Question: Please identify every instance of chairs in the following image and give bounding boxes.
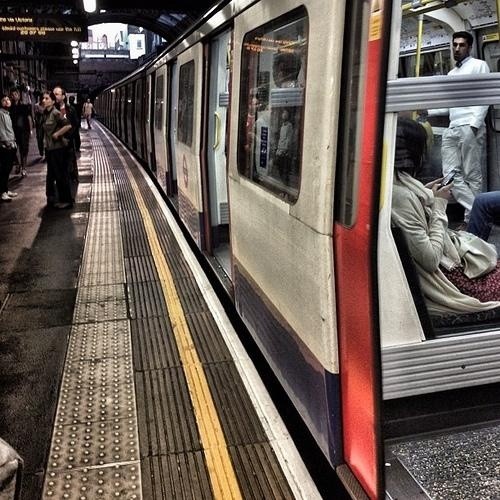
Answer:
[388,218,500,339]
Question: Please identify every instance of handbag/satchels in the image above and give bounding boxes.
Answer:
[62,140,76,161]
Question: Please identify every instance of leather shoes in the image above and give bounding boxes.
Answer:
[0,192,12,201]
[8,191,19,196]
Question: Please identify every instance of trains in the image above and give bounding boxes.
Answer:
[90,0,500,500]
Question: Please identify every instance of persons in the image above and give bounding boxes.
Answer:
[0,80,99,213]
[392,115,500,315]
[412,108,436,162]
[438,30,492,233]
[466,190,500,242]
[273,109,295,186]
[269,51,301,87]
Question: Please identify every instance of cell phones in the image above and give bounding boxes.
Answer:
[440,170,457,187]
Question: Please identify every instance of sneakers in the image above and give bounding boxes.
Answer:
[21,169,27,178]
[15,163,21,174]
[455,222,470,231]
